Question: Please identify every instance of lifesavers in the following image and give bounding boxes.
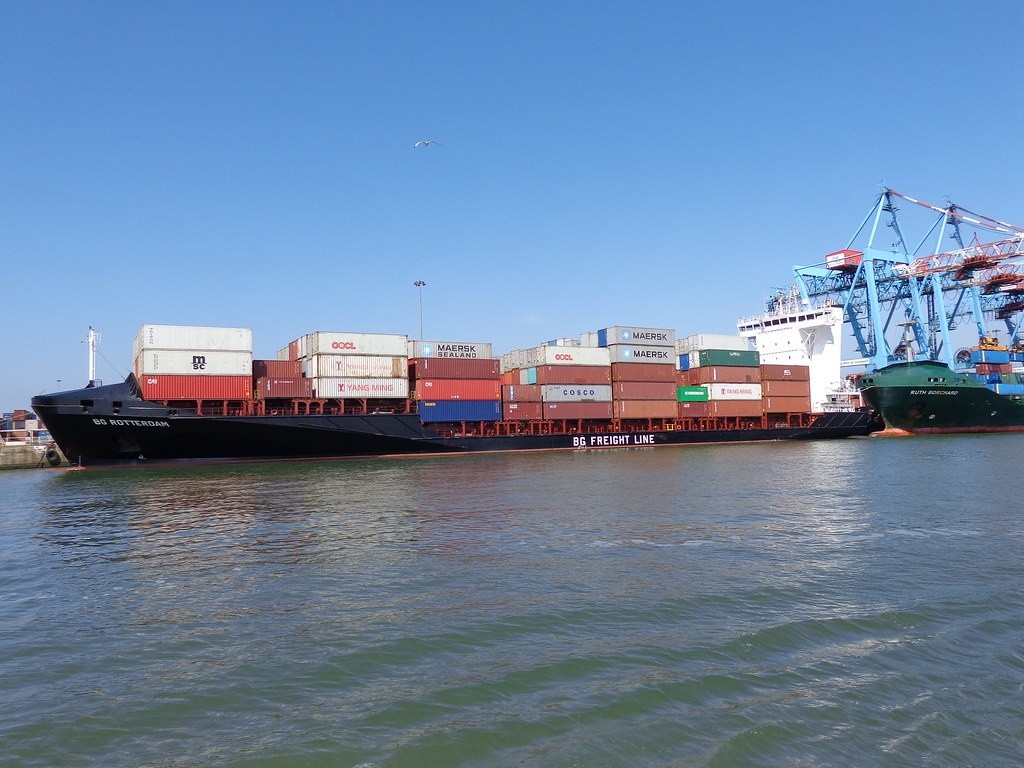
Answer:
[46,450,58,462]
[271,410,278,415]
[677,425,681,429]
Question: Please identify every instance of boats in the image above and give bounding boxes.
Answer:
[30,325,888,465]
[842,307,1024,433]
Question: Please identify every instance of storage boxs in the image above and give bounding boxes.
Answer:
[130,323,813,424]
[971,349,1024,398]
[825,249,878,271]
[0,409,50,440]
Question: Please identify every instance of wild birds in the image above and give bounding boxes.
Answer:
[414,140,441,149]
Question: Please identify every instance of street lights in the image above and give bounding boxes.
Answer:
[413,280,429,342]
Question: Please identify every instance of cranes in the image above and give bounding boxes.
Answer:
[761,174,1024,372]
